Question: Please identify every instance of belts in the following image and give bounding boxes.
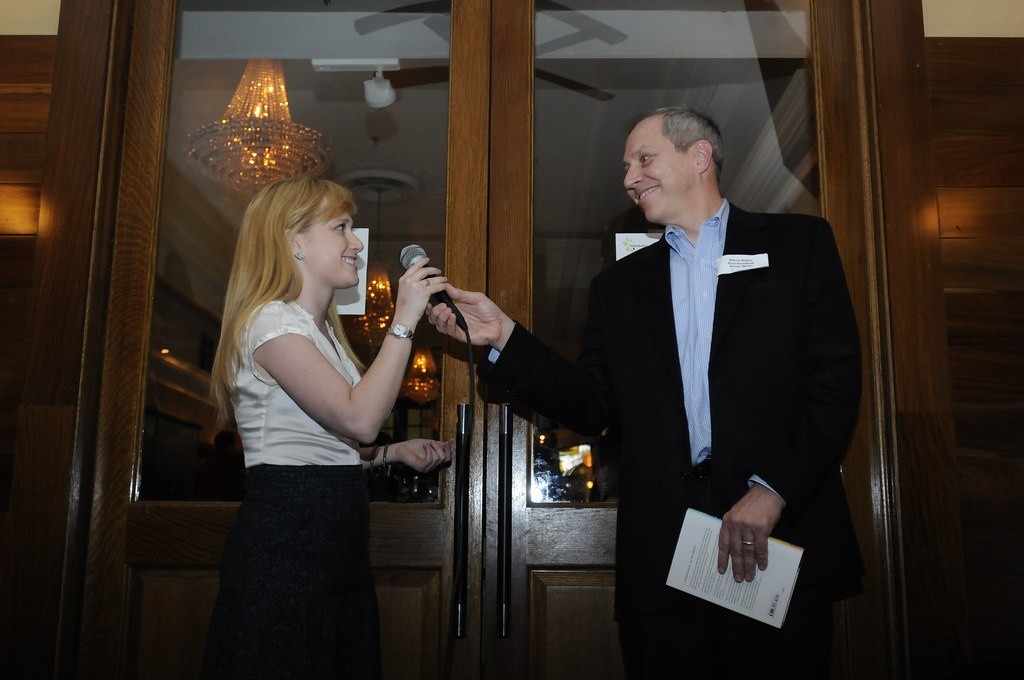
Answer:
[684,459,711,480]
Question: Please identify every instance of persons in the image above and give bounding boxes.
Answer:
[528,428,578,503]
[208,176,452,680]
[191,430,241,500]
[425,104,863,680]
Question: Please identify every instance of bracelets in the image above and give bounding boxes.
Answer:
[370,443,391,478]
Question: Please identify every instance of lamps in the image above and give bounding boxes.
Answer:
[340,170,422,347]
[405,345,439,406]
[364,67,398,110]
[183,59,334,194]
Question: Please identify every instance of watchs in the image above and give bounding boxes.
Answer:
[386,323,415,341]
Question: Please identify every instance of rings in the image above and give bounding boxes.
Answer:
[426,279,430,286]
[741,540,755,545]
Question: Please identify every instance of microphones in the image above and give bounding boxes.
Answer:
[400,244,469,331]
